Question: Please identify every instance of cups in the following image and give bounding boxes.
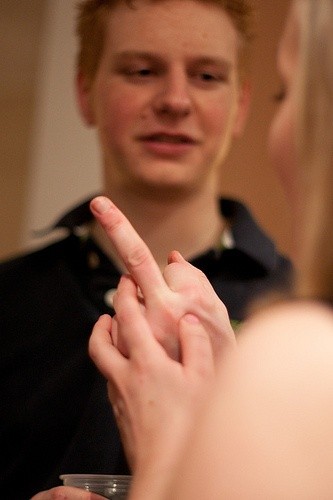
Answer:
[59,474,131,500]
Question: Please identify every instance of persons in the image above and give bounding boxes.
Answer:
[0,0,300,500]
[86,2,333,500]
